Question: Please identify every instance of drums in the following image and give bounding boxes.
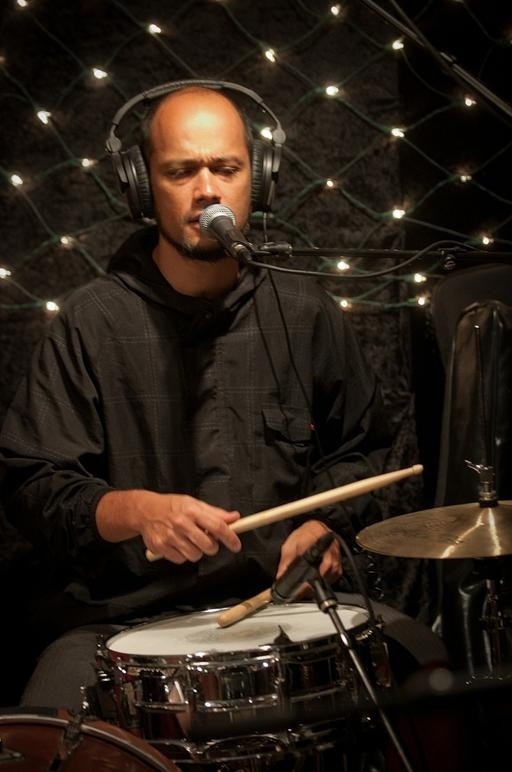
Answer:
[90,599,398,772]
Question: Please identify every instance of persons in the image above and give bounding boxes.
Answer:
[0,78,461,772]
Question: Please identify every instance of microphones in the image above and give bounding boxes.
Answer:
[270,531,336,605]
[199,203,253,263]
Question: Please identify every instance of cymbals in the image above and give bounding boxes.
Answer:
[356,500,512,559]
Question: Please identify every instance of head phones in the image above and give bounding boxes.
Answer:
[106,77,287,222]
